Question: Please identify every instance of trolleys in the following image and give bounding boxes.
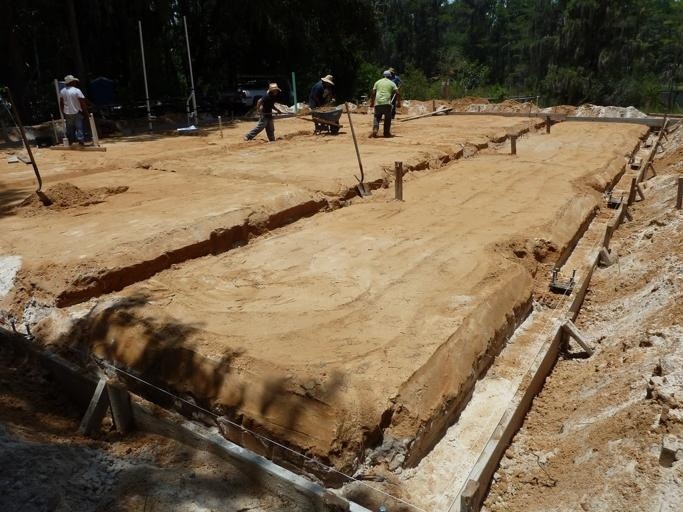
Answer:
[292,104,345,135]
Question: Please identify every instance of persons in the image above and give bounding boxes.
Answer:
[60,75,90,146]
[309,75,336,132]
[244,83,282,141]
[368,66,403,138]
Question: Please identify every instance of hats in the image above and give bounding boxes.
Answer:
[383,67,396,77]
[321,74,336,86]
[267,83,282,94]
[64,74,80,86]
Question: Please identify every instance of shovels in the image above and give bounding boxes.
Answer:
[6,88,52,206]
[345,102,371,197]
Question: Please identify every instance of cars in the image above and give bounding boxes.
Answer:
[218,78,278,110]
